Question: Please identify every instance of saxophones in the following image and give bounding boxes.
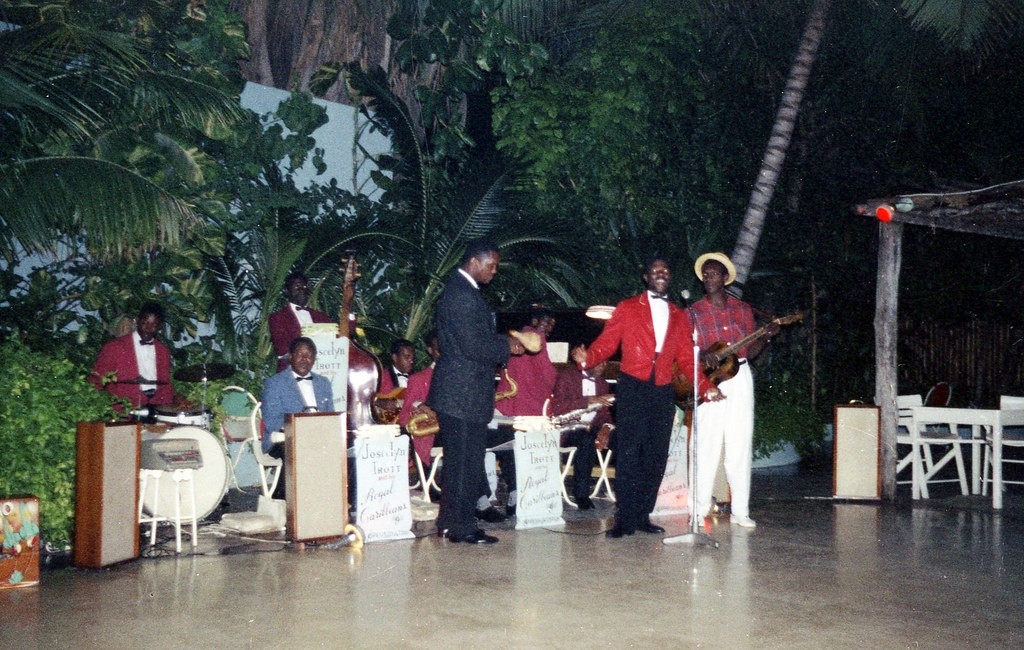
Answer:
[406,362,519,438]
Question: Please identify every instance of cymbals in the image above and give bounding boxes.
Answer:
[109,376,168,386]
[174,362,236,383]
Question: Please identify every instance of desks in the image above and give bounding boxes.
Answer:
[911,404,1024,507]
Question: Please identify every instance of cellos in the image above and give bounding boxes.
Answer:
[336,247,379,480]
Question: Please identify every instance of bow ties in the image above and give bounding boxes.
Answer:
[296,375,312,381]
[297,305,310,310]
[397,372,408,378]
[139,340,154,346]
[651,295,669,302]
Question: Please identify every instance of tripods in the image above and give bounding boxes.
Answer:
[661,321,719,549]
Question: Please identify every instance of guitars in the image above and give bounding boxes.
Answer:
[670,306,804,413]
[372,355,431,426]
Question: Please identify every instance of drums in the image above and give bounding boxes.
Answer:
[152,400,214,431]
[140,425,230,525]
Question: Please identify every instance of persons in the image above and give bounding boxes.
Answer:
[69,234,816,607]
[546,358,615,513]
[425,239,528,547]
[373,340,421,492]
[572,256,725,538]
[494,309,558,416]
[84,309,197,421]
[259,336,353,510]
[682,248,780,528]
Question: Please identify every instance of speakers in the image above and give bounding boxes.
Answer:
[833,403,881,498]
[77,418,141,567]
[284,412,349,542]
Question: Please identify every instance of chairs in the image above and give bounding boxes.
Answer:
[898,395,1024,508]
[216,386,616,506]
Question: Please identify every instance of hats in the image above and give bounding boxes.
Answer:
[696,251,737,286]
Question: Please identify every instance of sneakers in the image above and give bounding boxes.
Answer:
[475,505,505,523]
[449,527,497,545]
[438,527,452,535]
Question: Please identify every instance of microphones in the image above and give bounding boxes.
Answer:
[681,290,695,320]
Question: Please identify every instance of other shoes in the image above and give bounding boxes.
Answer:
[505,503,516,517]
[688,514,704,527]
[729,514,755,529]
[576,496,596,512]
[638,522,666,533]
[605,522,636,539]
